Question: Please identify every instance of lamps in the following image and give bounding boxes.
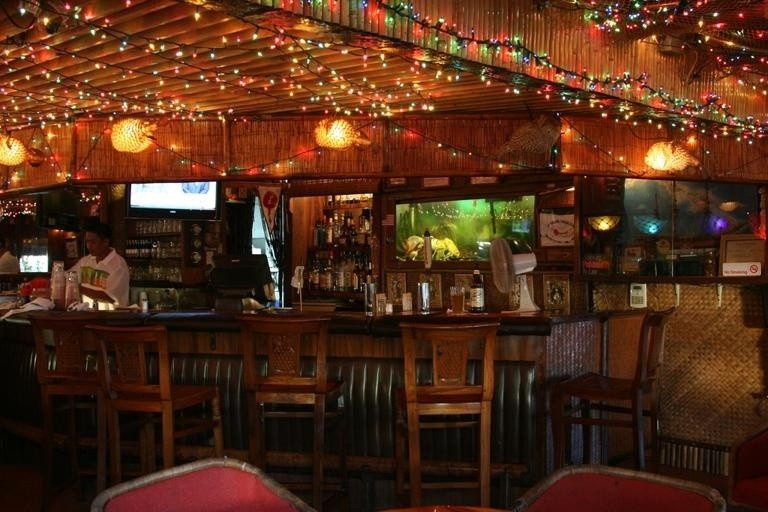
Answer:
[314,118,371,153]
[642,141,701,174]
[632,215,669,235]
[0,137,26,168]
[587,215,621,232]
[111,115,158,153]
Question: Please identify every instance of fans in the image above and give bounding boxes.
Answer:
[489,237,541,314]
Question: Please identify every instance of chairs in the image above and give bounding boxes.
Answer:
[550,305,676,471]
[25,313,120,495]
[87,457,318,512]
[509,464,727,512]
[82,323,223,489]
[238,315,349,510]
[392,320,499,508]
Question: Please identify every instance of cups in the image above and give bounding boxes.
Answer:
[65,271,80,307]
[450,287,465,313]
[51,262,65,310]
[417,283,430,314]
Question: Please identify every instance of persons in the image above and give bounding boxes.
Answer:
[49,224,129,307]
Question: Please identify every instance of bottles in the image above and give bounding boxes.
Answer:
[365,297,394,318]
[138,289,176,313]
[403,292,412,311]
[125,219,182,281]
[470,269,486,312]
[307,194,372,291]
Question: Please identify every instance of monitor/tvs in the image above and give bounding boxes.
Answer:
[213,254,273,287]
[127,180,220,219]
